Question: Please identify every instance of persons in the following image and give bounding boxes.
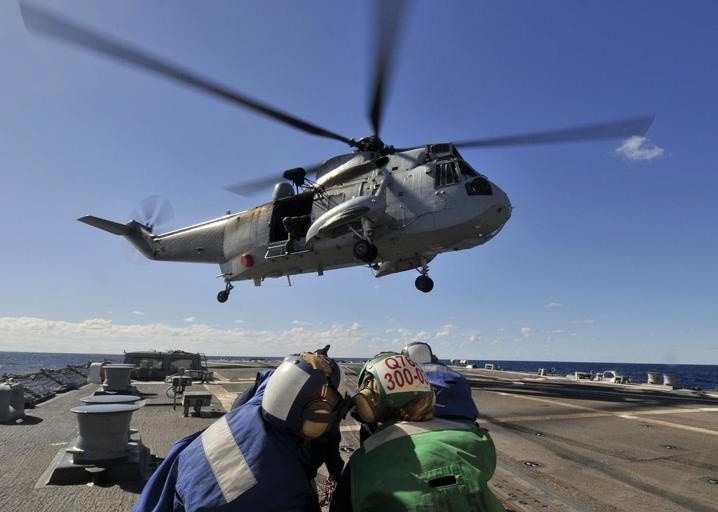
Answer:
[131,341,342,512]
[328,349,507,512]
[401,341,480,421]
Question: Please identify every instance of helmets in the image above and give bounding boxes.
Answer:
[358,352,431,407]
[401,343,433,364]
[261,353,340,432]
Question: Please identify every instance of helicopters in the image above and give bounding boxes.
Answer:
[19,1,654,303]
